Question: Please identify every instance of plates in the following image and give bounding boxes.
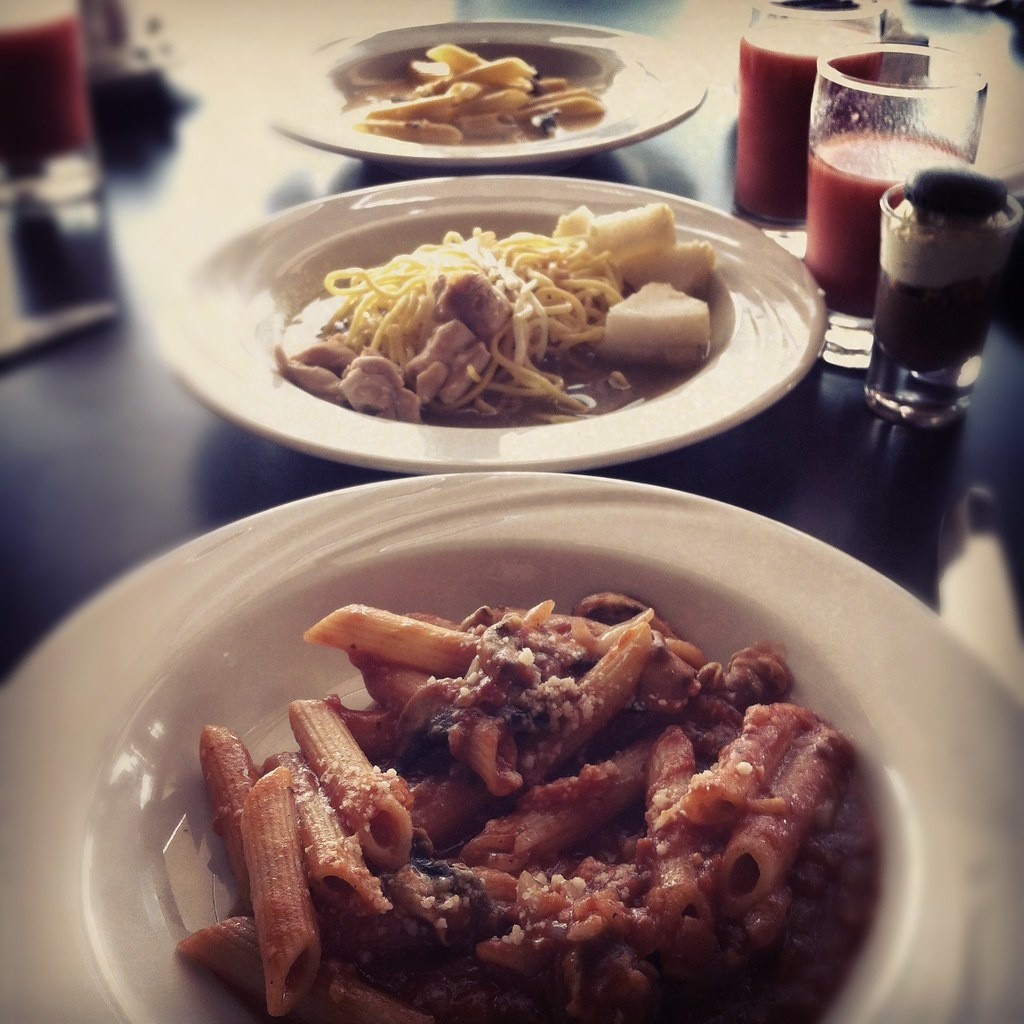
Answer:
[0,472,1024,1024]
[160,176,827,473]
[265,21,707,164]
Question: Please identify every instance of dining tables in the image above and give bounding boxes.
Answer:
[1,0,1023,673]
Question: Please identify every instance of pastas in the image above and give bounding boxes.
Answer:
[177,592,872,1024]
[353,40,608,143]
[318,226,635,423]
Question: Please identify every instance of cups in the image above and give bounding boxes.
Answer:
[1,1,105,205]
[803,44,987,368]
[735,1,887,220]
[864,181,1024,425]
